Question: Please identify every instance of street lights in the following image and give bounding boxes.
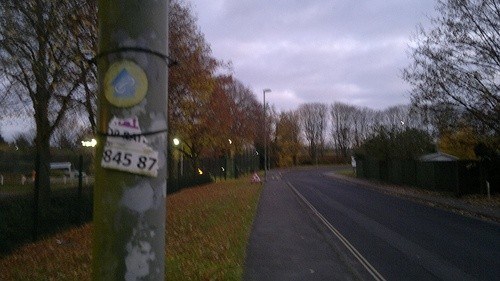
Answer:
[263,89,272,178]
